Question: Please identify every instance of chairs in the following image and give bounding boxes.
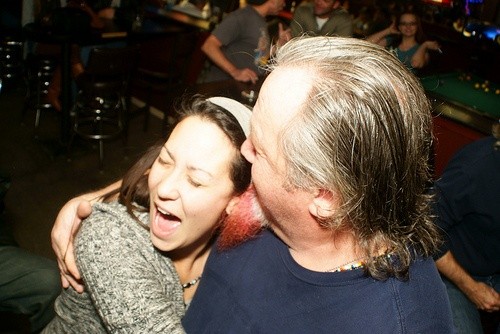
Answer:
[7,31,199,162]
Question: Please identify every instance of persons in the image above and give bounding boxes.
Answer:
[50,37,453,334]
[57,0,443,117]
[415,127,496,333]
[42,97,252,334]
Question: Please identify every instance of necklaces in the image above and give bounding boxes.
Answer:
[182,275,204,291]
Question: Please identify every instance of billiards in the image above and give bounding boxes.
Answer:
[439,73,500,94]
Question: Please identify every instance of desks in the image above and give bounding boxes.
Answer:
[23,22,146,162]
[420,70,500,177]
